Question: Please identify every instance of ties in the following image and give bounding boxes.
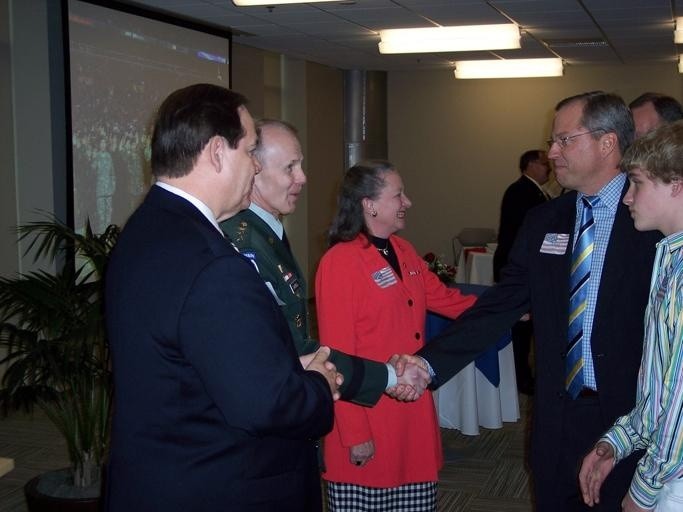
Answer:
[563,195,601,401]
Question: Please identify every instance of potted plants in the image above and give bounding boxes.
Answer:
[0,209,122,511]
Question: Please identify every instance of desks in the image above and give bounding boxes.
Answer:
[422,242,524,434]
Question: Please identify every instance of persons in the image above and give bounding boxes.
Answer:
[216,118,434,511]
[577,118,683,511]
[312,158,531,512]
[68,47,162,234]
[626,90,682,136]
[100,82,347,512]
[385,89,667,511]
[492,147,558,397]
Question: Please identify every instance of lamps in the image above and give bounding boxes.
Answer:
[377,24,566,80]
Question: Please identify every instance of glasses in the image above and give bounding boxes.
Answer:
[545,128,607,152]
